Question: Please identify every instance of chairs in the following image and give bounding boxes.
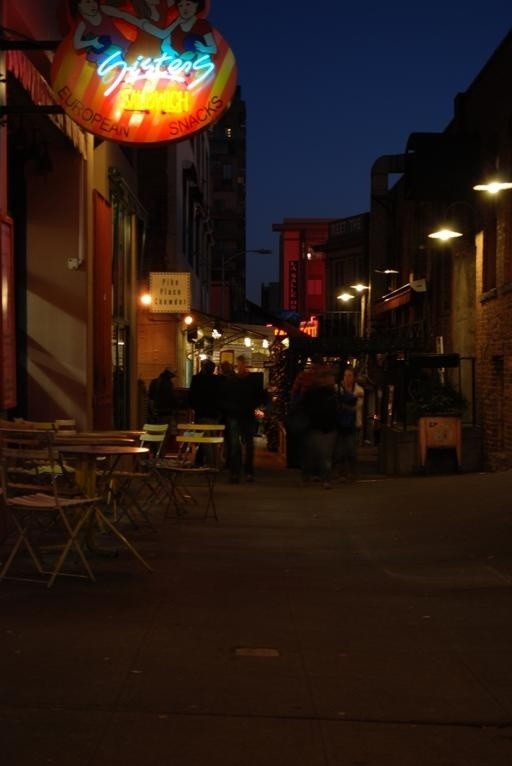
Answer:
[0,418,227,589]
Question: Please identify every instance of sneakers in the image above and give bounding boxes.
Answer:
[229,473,258,483]
[302,472,353,489]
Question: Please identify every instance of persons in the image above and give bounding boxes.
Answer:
[136,0,217,71]
[136,345,419,485]
[68,0,152,67]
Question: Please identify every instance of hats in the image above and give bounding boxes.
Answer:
[165,365,179,376]
